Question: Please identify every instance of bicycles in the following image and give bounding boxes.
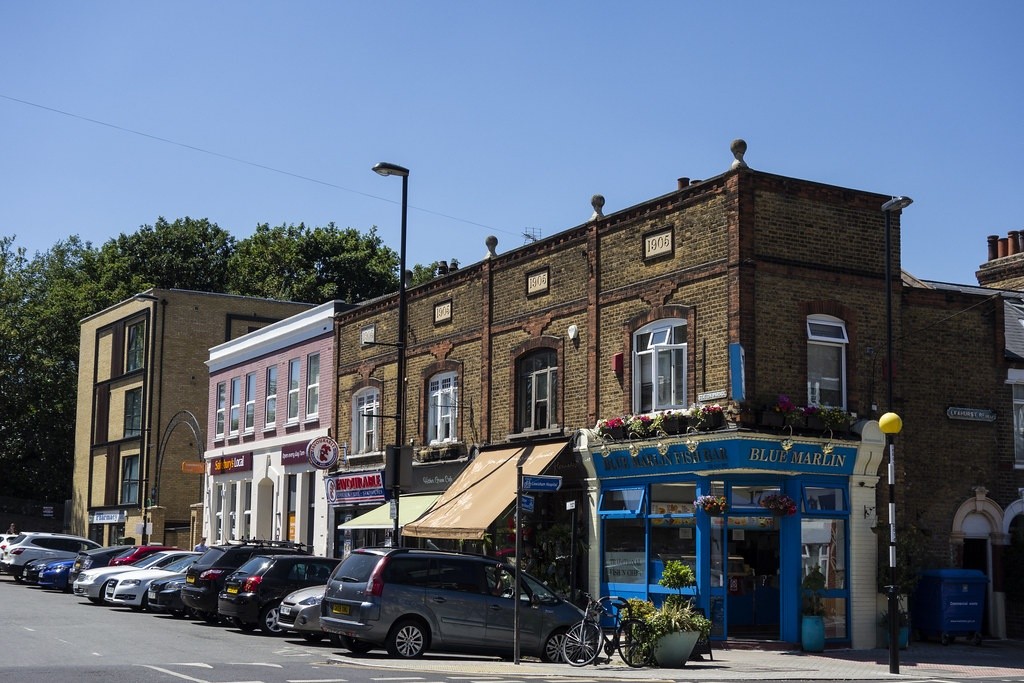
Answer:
[560,588,654,668]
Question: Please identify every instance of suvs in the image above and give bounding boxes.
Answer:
[108,545,184,567]
[217,555,342,637]
[318,546,595,664]
[1,531,104,584]
[67,545,133,586]
[180,540,315,629]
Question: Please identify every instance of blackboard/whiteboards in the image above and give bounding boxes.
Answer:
[692,608,711,654]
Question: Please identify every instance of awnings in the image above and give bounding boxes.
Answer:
[400,442,568,540]
[337,494,443,529]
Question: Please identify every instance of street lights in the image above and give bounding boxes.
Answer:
[370,160,410,551]
[132,293,166,507]
[881,194,914,673]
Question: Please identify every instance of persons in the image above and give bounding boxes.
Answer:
[6,523,17,535]
[524,546,537,576]
[194,538,208,552]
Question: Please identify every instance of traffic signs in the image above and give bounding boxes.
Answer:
[521,474,562,492]
[521,494,535,513]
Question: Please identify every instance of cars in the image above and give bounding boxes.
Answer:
[0,534,19,561]
[20,558,67,584]
[72,551,205,607]
[103,554,202,612]
[148,573,206,621]
[275,584,326,644]
[37,559,77,594]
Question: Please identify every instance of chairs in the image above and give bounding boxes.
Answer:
[307,566,318,579]
[318,568,328,581]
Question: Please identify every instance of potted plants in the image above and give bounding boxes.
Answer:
[801,564,827,653]
[878,609,911,650]
[641,560,714,668]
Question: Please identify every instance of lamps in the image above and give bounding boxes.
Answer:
[628,432,640,457]
[601,433,615,457]
[876,196,913,215]
[781,424,794,452]
[656,430,669,456]
[685,426,699,452]
[820,427,834,454]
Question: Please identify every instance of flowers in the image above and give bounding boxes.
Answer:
[598,417,625,428]
[690,404,721,424]
[627,415,652,436]
[694,494,727,511]
[648,411,684,440]
[758,494,797,517]
[762,394,852,432]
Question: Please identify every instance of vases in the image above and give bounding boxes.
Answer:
[804,416,841,432]
[625,419,655,440]
[759,413,792,428]
[656,416,687,436]
[602,425,630,440]
[686,412,720,431]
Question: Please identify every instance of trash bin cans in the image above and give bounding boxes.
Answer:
[993,592,1007,640]
[909,569,991,646]
